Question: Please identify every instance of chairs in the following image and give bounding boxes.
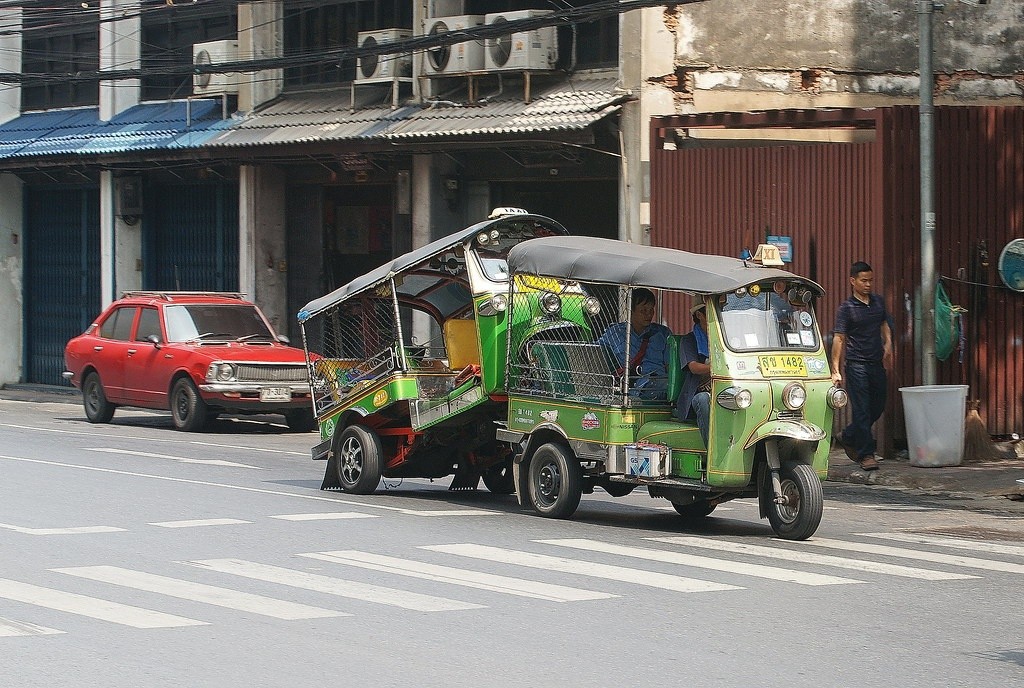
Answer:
[666,335,698,424]
[443,318,480,370]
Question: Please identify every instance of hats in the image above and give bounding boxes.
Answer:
[688,295,706,314]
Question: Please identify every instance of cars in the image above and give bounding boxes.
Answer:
[62,291,330,431]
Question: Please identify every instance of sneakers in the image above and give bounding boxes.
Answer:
[859,454,880,471]
[835,432,858,462]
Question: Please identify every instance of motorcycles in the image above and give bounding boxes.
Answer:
[294,211,601,496]
[495,235,849,540]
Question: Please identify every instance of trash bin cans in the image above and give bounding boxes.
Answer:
[899,385,971,469]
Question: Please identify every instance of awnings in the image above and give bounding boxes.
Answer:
[200,77,625,147]
[0,99,244,158]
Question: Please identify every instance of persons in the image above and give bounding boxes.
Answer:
[829,262,893,470]
[594,288,673,400]
[675,295,711,451]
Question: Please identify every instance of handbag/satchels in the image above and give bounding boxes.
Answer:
[610,367,629,395]
[935,283,969,361]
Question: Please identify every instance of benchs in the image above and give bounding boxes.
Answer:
[315,358,450,406]
[527,340,680,424]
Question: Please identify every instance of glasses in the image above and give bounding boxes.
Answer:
[633,306,654,314]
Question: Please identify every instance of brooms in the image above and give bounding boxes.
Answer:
[965,284,1010,463]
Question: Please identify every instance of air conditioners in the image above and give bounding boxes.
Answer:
[421,14,486,74]
[355,29,412,79]
[485,9,558,67]
[192,40,239,95]
